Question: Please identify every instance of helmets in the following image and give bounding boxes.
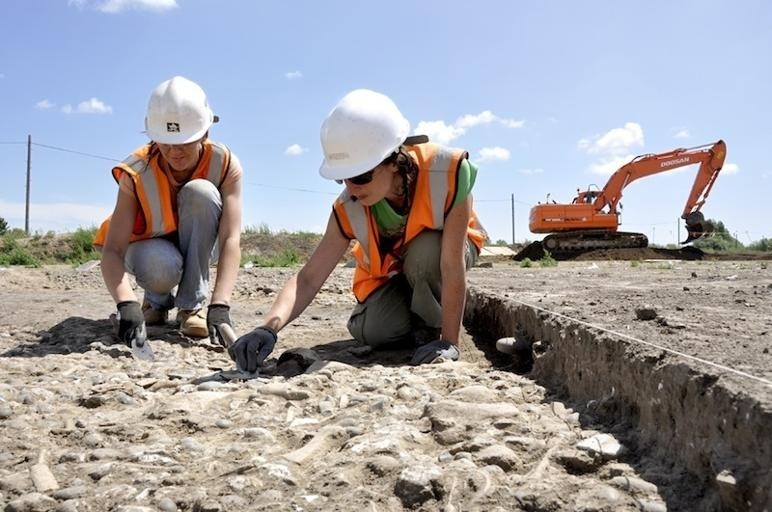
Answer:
[144,76,214,146]
[319,88,410,182]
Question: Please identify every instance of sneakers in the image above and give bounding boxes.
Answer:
[141,295,168,326]
[175,306,209,338]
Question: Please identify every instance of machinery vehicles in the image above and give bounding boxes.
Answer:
[528,140,726,254]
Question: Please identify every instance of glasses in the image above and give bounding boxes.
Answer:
[334,169,374,186]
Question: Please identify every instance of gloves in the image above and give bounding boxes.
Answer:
[116,300,148,348]
[408,340,460,366]
[206,303,238,350]
[227,325,278,375]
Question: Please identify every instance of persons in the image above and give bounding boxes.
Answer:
[93,75,243,349]
[228,87,489,375]
